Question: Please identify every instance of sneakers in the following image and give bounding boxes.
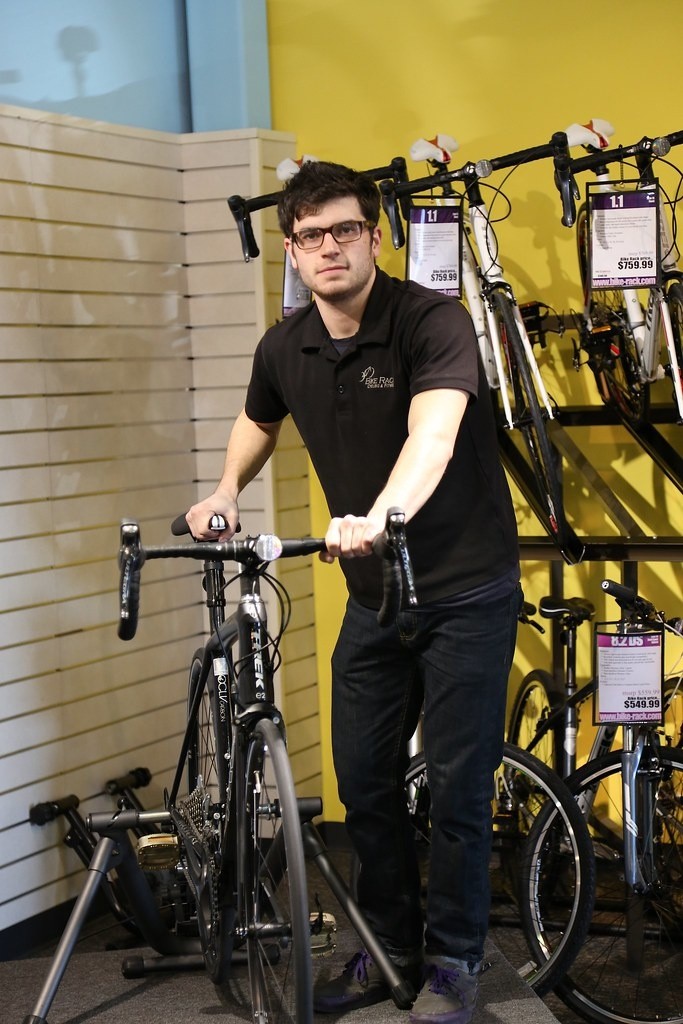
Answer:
[313,950,424,1013]
[409,962,480,1024]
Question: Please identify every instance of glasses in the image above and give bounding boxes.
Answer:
[289,220,376,250]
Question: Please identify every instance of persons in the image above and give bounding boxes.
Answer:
[184,160,521,1024]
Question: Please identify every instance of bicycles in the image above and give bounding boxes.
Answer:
[504,579,683,1024]
[227,119,682,533]
[118,510,420,1024]
